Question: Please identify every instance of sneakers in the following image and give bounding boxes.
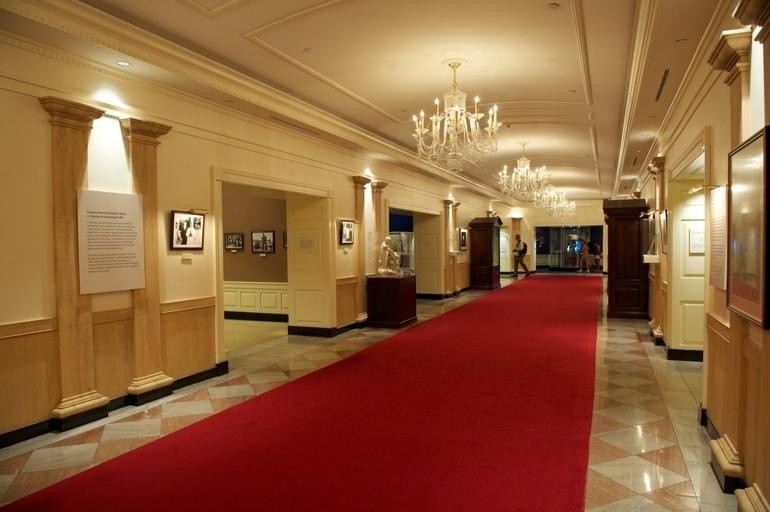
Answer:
[576,269,591,272]
[511,274,518,277]
[524,273,529,278]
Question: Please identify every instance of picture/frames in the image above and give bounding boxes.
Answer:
[340,219,354,244]
[170,209,204,252]
[224,232,243,250]
[459,228,467,250]
[250,230,276,254]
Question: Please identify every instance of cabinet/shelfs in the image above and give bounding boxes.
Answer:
[601,199,651,320]
[469,217,504,290]
[724,126,770,331]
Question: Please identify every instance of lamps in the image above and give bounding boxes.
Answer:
[412,56,503,176]
[496,141,576,219]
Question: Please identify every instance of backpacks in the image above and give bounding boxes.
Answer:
[520,243,526,255]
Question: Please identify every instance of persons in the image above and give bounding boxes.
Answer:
[576,239,603,273]
[185,220,197,246]
[379,237,403,275]
[511,234,531,277]
[261,234,268,250]
[180,220,192,245]
